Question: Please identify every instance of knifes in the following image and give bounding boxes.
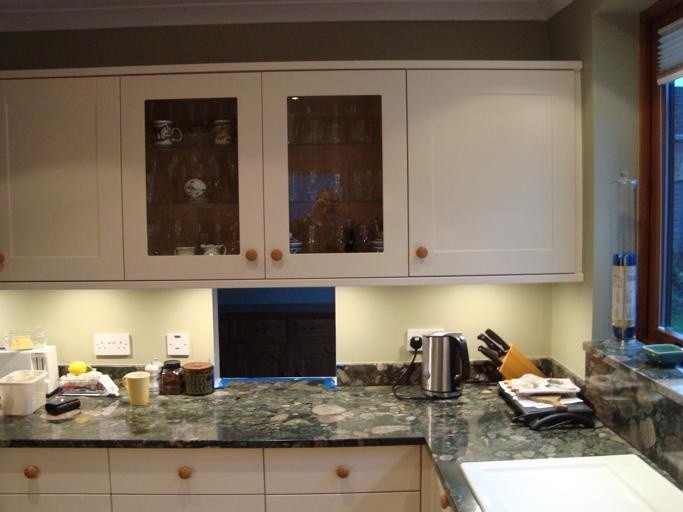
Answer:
[477,328,511,367]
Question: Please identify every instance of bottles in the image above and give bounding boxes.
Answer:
[601,170,645,352]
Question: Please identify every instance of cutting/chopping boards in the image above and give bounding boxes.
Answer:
[503,379,569,412]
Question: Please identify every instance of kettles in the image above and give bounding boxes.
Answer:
[421,331,471,400]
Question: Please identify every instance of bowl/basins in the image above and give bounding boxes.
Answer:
[642,341,683,368]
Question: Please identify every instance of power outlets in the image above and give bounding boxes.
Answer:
[93,334,132,356]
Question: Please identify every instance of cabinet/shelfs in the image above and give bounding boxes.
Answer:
[264,448,422,511]
[0,67,123,292]
[119,61,408,292]
[108,448,266,511]
[422,448,457,511]
[407,61,584,285]
[1,447,111,511]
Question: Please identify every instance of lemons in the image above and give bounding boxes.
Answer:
[67,361,87,375]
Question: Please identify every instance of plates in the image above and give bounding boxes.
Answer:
[511,374,582,394]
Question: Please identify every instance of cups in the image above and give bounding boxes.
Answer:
[122,370,151,407]
[152,119,184,150]
[212,119,234,147]
[173,246,197,256]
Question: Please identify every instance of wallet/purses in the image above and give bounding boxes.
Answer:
[44,397,81,414]
